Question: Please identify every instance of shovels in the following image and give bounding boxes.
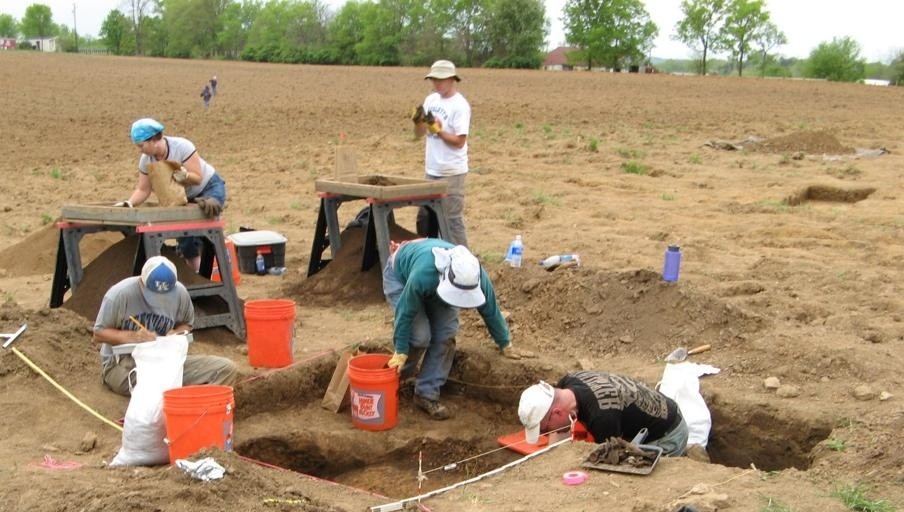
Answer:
[663,344,713,362]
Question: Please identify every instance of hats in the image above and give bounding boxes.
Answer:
[435,246,486,309]
[141,256,177,308]
[519,380,556,445]
[424,60,457,80]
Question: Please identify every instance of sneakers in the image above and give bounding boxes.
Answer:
[413,393,451,420]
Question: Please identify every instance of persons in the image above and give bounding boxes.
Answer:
[382,236,536,421]
[209,75,218,96]
[518,369,689,458]
[92,254,238,397]
[111,117,227,275]
[411,59,472,250]
[200,84,212,107]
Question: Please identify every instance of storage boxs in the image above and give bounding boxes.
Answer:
[227,230,288,274]
[198,234,241,286]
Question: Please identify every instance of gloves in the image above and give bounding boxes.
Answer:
[423,111,441,134]
[412,106,426,128]
[503,341,536,358]
[195,196,223,217]
[172,166,189,183]
[176,457,227,482]
[111,200,133,208]
[386,351,408,373]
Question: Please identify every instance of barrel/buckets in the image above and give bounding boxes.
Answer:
[210,239,241,286]
[163,384,235,465]
[244,299,297,369]
[347,354,399,430]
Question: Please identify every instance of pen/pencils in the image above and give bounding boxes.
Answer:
[129,316,146,330]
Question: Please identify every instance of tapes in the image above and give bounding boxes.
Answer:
[562,472,589,485]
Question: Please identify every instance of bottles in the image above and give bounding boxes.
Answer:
[505,234,523,268]
[255,251,264,275]
[662,243,681,283]
[539,254,579,268]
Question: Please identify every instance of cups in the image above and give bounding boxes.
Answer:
[268,267,281,275]
